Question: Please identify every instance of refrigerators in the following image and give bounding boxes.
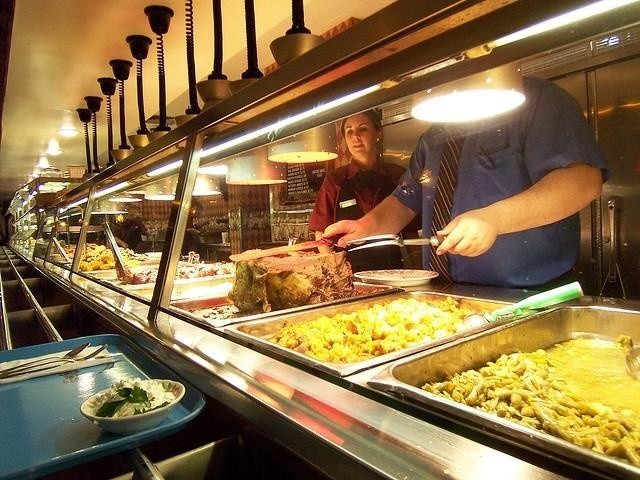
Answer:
[516,26,639,301]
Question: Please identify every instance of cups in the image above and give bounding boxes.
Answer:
[221,231,228,243]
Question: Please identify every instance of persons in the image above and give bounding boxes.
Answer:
[113,205,148,251]
[323,76,612,288]
[308,109,422,272]
[181,199,204,255]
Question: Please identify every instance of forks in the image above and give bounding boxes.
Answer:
[0,343,109,381]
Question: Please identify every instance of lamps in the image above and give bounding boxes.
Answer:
[67,2,289,217]
[265,1,340,166]
[408,58,527,124]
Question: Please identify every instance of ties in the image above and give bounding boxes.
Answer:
[430,132,468,280]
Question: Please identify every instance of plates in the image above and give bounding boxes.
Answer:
[354,269,440,288]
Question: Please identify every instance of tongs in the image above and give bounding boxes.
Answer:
[103,221,130,283]
[52,237,72,268]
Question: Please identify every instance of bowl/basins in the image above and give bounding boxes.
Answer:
[79,378,187,434]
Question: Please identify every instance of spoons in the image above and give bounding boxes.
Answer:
[0,341,91,374]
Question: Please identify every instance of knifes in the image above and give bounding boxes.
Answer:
[229,233,345,262]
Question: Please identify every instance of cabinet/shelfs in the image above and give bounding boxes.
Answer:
[377,100,429,272]
[5,2,640,478]
[590,33,639,306]
[514,36,602,303]
